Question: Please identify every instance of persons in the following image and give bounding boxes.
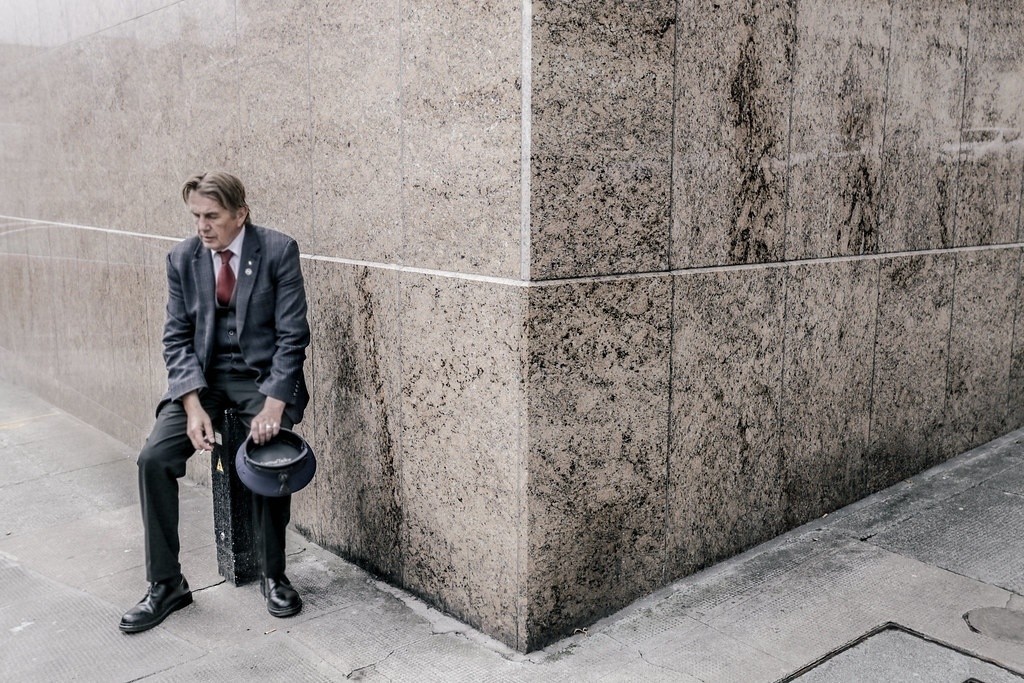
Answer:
[119,167,310,631]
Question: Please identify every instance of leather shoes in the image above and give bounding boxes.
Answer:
[260,571,303,617]
[119,573,193,632]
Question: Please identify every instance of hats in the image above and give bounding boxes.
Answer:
[234,428,318,499]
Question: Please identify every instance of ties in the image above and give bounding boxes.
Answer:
[215,250,236,306]
